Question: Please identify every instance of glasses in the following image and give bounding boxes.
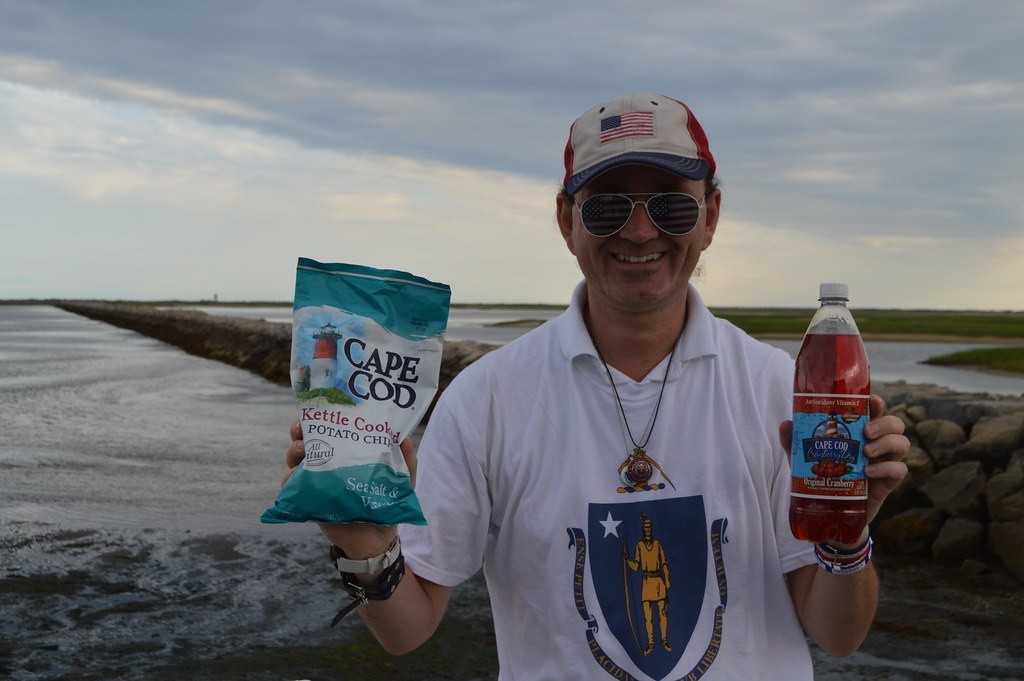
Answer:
[570,190,707,239]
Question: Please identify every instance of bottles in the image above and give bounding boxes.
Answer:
[788,283,872,541]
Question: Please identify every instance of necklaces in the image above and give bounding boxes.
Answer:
[582,299,691,487]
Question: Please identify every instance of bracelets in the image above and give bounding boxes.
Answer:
[328,534,405,628]
[815,533,872,575]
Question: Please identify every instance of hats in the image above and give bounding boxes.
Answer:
[564,95,717,188]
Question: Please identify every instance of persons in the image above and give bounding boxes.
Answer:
[280,95,910,681]
